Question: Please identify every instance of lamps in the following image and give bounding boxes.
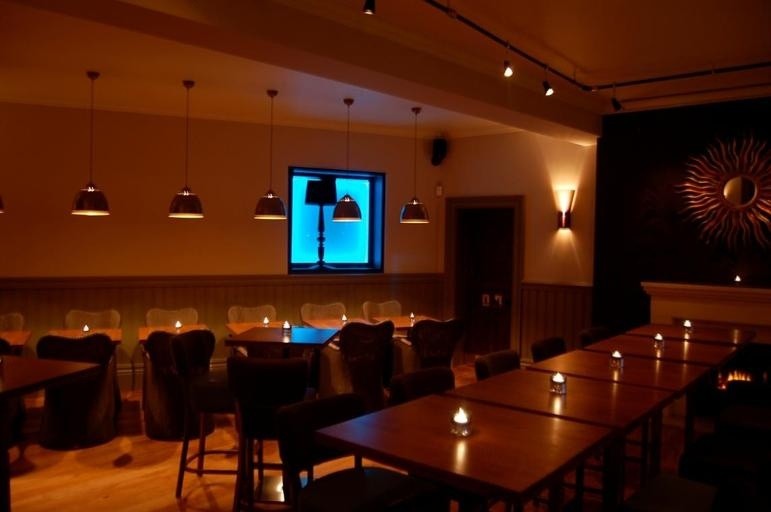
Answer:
[557,190,575,229]
[305,181,338,271]
[253,89,288,220]
[167,80,204,219]
[333,99,361,221]
[401,107,430,224]
[363,0,771,115]
[71,70,109,216]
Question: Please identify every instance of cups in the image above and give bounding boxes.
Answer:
[79,313,417,336]
[450,318,697,439]
